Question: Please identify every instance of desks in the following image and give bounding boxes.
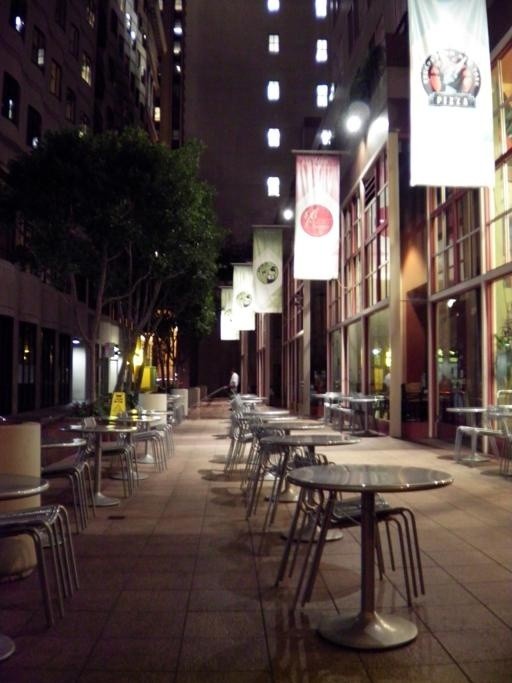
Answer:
[446,407,489,462]
[286,466,453,649]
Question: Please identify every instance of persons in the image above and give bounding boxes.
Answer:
[382,367,391,396]
[227,368,239,398]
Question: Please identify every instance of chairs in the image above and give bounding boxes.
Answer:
[454,390,512,476]
